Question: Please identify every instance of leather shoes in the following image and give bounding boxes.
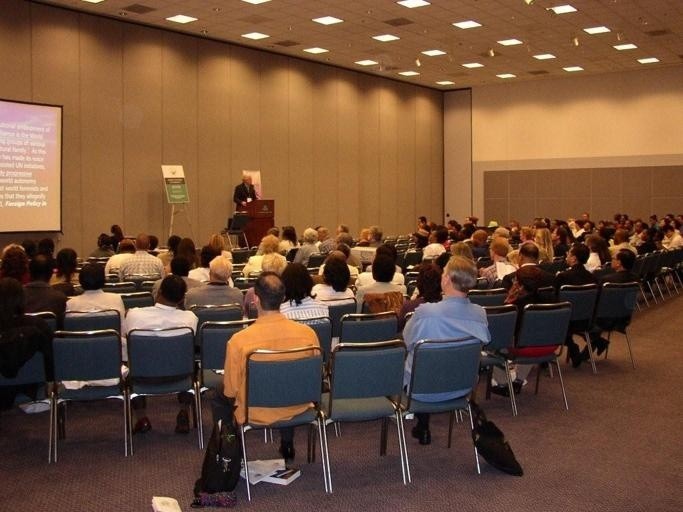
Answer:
[569,336,609,368]
[176,409,189,432]
[492,385,509,396]
[412,426,431,445]
[135,417,150,433]
[512,379,522,393]
[279,446,295,464]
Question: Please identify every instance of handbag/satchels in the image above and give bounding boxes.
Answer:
[472,410,523,477]
[193,425,242,498]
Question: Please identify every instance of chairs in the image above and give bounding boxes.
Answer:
[221,211,250,251]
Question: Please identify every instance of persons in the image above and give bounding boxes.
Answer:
[220,274,319,465]
[1,210,683,431]
[397,254,489,447]
[234,176,258,206]
